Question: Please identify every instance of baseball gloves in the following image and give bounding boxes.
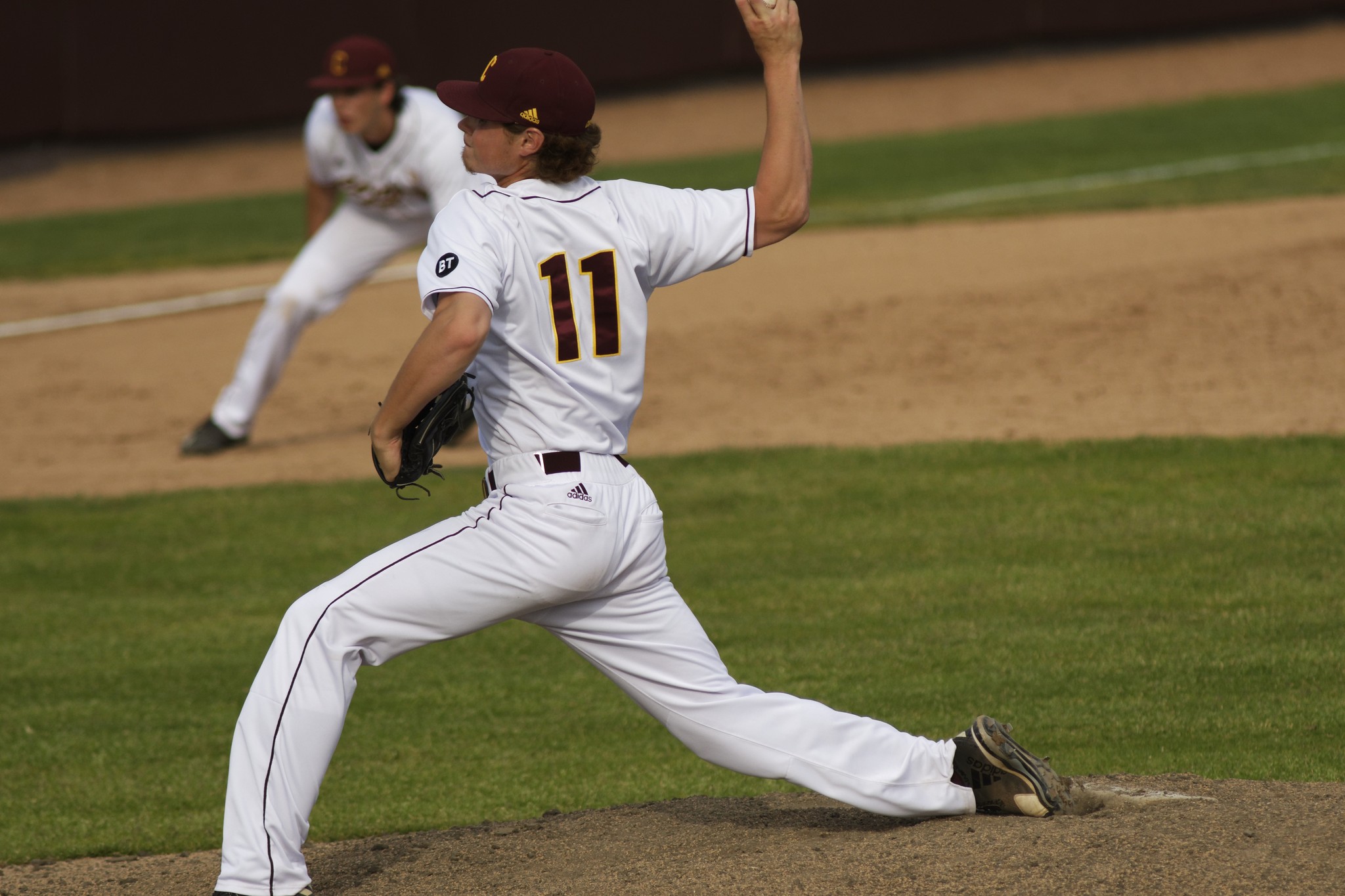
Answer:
[365,370,476,505]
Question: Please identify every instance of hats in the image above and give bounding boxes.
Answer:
[436,48,594,139]
[305,35,389,90]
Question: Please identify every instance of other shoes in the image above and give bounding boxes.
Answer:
[178,415,249,457]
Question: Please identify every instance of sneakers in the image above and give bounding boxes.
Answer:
[950,716,1078,814]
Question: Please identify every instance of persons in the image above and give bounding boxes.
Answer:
[179,37,497,455]
[211,0,1104,895]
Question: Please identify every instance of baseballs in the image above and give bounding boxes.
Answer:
[746,0,778,11]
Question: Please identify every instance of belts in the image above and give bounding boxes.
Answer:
[482,450,632,492]
